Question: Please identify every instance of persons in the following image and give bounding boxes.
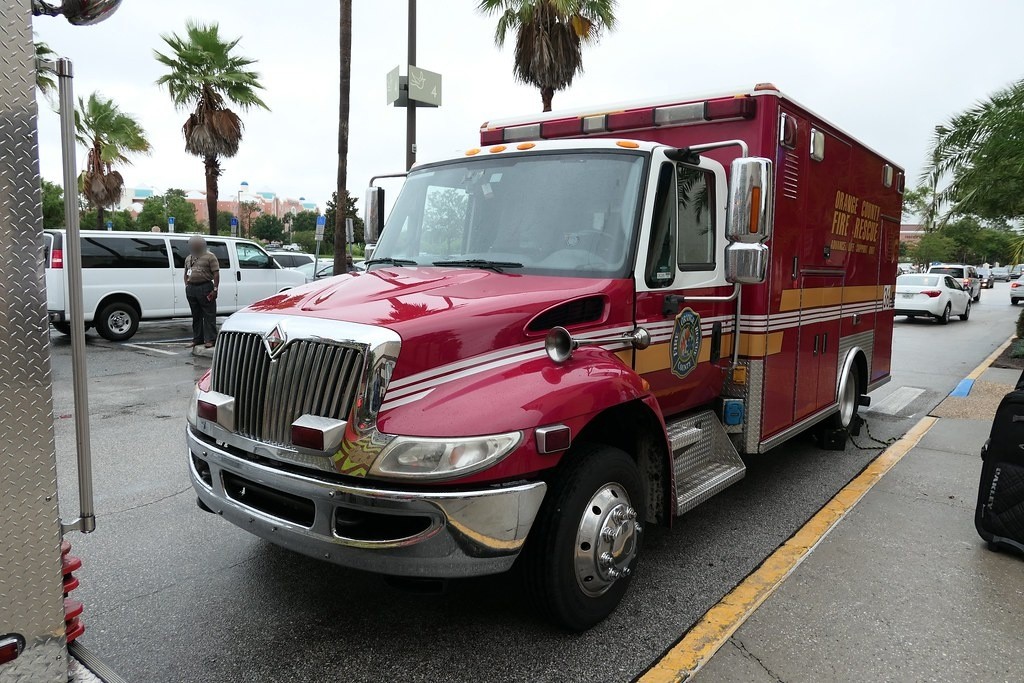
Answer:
[183,236,220,348]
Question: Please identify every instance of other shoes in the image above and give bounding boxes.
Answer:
[185,340,204,348]
[205,340,215,348]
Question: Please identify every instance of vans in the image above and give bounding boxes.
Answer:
[927,265,981,302]
[43,230,306,344]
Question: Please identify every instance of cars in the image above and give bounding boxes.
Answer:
[976,268,994,289]
[1010,275,1024,306]
[1018,265,1024,272]
[895,273,973,326]
[991,268,1011,282]
[897,263,925,276]
[1005,266,1014,273]
[1007,266,1022,279]
[313,263,365,283]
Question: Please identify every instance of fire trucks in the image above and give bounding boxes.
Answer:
[182,81,908,636]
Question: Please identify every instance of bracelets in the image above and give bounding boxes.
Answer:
[213,287,218,292]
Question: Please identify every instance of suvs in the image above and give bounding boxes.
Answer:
[248,256,317,284]
